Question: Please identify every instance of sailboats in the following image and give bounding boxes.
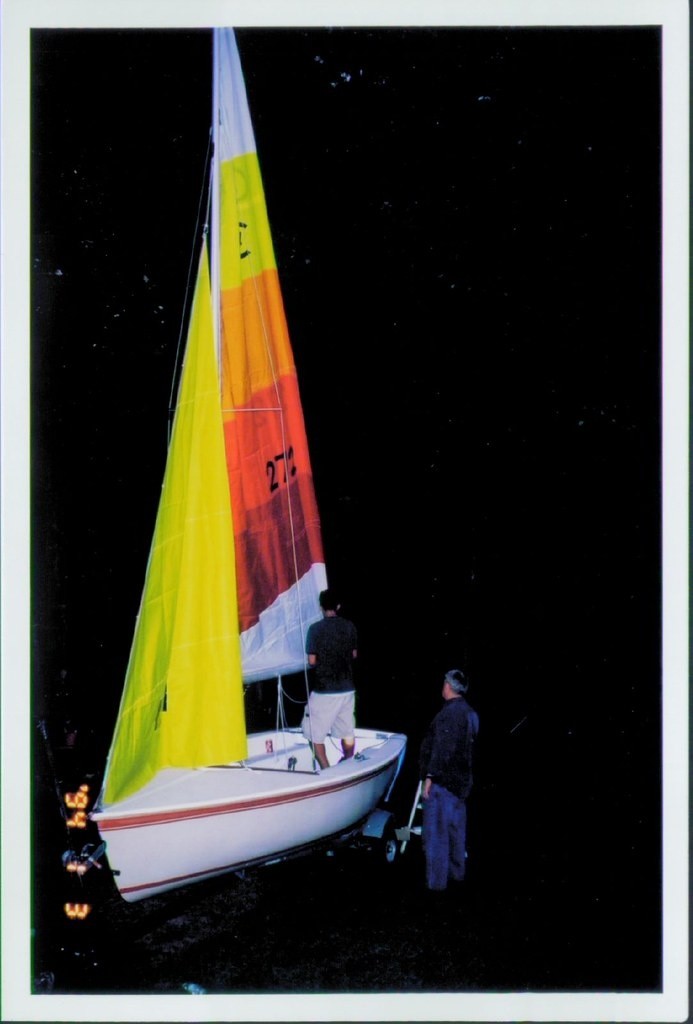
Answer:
[75,29,410,907]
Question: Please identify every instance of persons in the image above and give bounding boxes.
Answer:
[417,666,482,893]
[303,587,358,770]
